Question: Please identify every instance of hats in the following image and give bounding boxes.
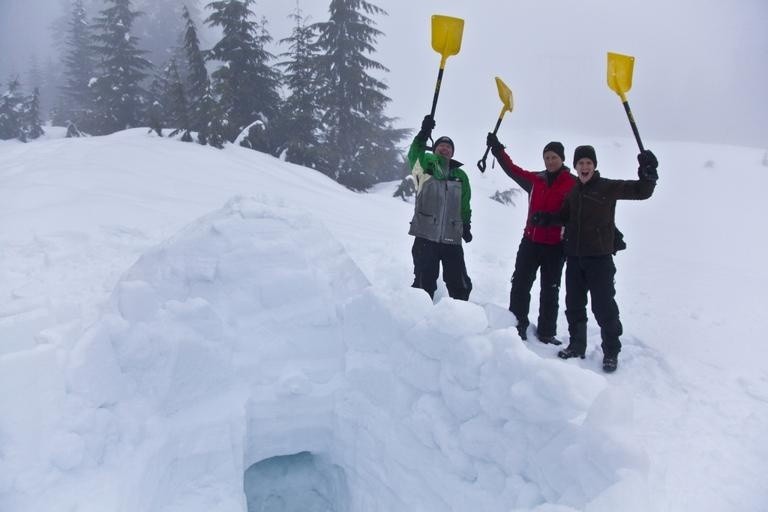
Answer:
[542,141,565,162]
[572,145,597,169]
[433,136,454,156]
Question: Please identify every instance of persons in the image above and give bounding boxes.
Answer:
[407,114,473,302]
[485,132,577,346]
[528,145,660,372]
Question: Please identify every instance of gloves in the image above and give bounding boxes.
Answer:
[417,115,436,141]
[637,150,659,181]
[462,224,473,243]
[486,132,500,148]
[531,211,556,226]
[612,227,626,256]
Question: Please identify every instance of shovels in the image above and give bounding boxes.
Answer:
[607,52,659,180]
[477,77,513,172]
[419,15,464,151]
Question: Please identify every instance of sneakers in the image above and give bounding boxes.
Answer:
[601,351,618,373]
[507,317,587,359]
